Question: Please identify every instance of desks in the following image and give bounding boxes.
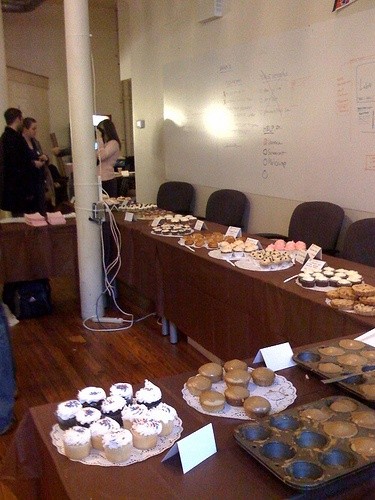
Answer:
[0,208,375,500]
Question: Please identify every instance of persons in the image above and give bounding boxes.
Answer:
[0,108,71,218]
[96,119,121,197]
[0,260,19,435]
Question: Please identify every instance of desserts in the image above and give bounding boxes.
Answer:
[56,379,174,463]
[107,196,197,235]
[301,337,375,457]
[218,239,306,268]
[187,359,276,419]
[297,266,364,288]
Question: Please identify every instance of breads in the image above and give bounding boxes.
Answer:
[183,232,246,248]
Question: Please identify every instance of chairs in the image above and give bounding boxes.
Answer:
[157,181,196,216]
[339,218,375,267]
[252,200,345,255]
[194,189,251,232]
[125,156,136,190]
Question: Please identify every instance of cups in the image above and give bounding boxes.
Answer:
[118,168,122,174]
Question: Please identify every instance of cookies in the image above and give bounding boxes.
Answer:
[326,283,375,316]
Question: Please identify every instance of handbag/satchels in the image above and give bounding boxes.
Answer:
[3,277,53,320]
